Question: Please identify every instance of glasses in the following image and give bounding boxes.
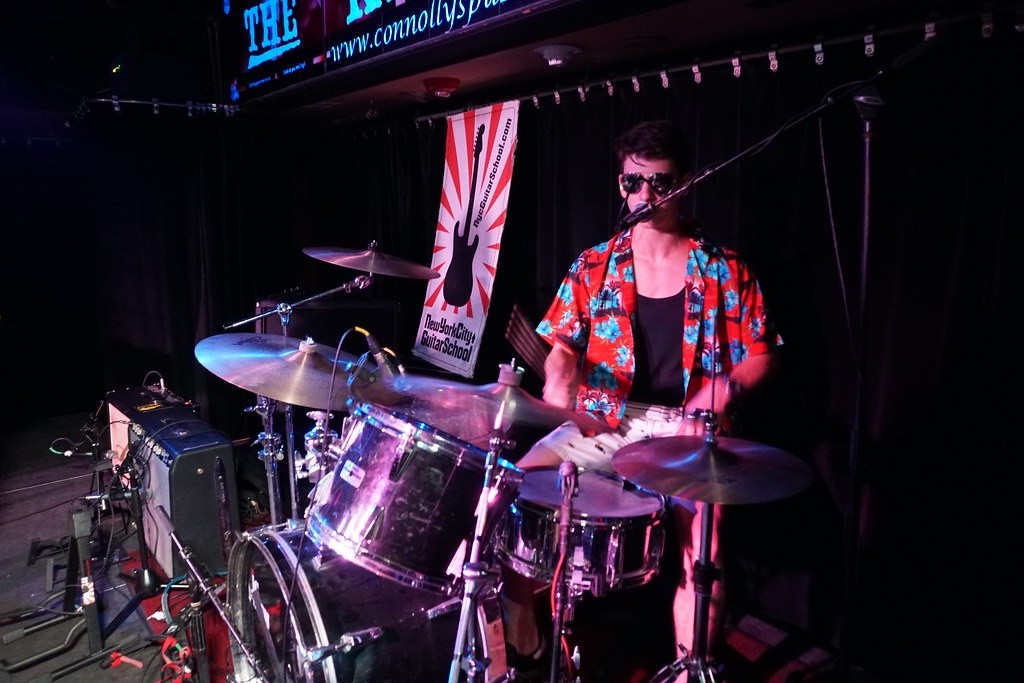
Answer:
[621,171,680,198]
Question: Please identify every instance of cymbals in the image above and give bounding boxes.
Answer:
[301,240,443,280]
[192,331,378,415]
[608,431,816,508]
[389,358,595,431]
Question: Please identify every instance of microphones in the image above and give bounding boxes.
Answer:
[76,490,134,501]
[619,201,648,232]
[63,450,94,455]
[366,334,396,379]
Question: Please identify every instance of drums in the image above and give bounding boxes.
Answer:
[488,463,667,595]
[227,518,513,683]
[303,400,527,599]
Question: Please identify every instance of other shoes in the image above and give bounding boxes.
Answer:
[471,630,561,683]
[674,658,726,683]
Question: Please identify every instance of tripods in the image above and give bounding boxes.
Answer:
[653,503,718,683]
[99,435,193,647]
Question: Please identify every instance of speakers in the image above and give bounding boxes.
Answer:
[254,299,402,410]
[106,387,241,582]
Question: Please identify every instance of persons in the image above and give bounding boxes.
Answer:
[492,121,786,683]
